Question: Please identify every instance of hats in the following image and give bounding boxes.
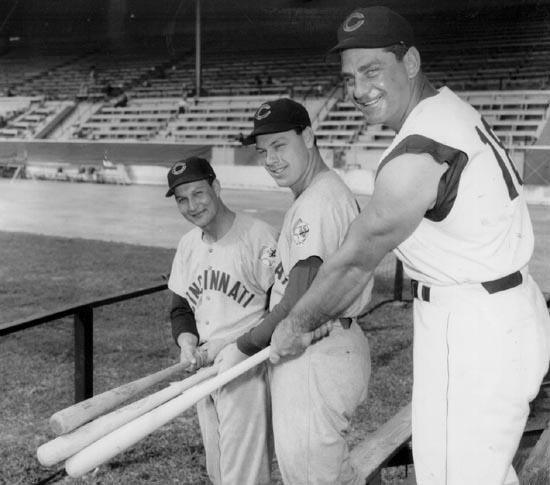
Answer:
[326,5,415,55]
[165,155,216,198]
[242,98,312,146]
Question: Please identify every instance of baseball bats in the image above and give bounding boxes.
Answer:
[65,321,334,479]
[51,360,192,436]
[36,365,221,467]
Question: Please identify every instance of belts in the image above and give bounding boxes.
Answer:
[336,316,352,331]
[412,270,523,302]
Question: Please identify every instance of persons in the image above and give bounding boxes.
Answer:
[167,156,280,485]
[167,6,550,485]
[270,8,550,485]
[218,98,373,484]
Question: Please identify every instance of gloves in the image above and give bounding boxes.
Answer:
[213,327,262,377]
